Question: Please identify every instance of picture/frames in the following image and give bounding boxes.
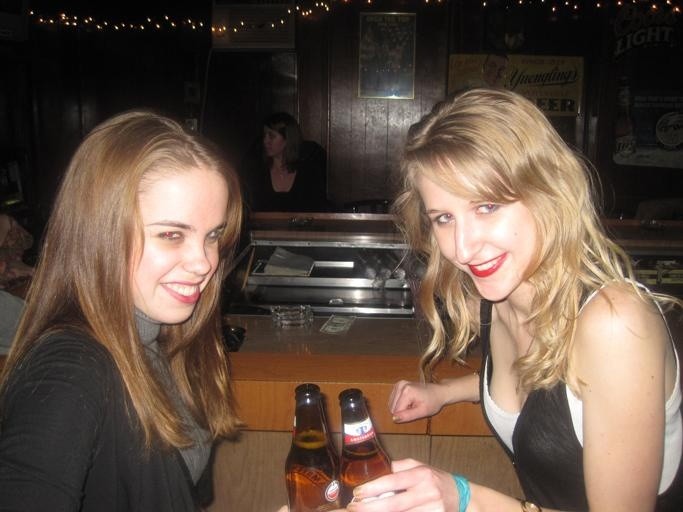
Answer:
[355,10,415,101]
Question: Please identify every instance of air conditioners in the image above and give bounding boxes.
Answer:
[208,0,296,51]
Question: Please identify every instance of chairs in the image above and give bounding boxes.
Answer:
[344,199,393,212]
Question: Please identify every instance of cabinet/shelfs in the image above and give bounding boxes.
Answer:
[222,213,682,318]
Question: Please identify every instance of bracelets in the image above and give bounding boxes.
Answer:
[513,495,543,512]
[452,471,470,511]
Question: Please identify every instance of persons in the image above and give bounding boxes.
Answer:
[240,117,322,213]
[0,110,347,511]
[53,107,98,187]
[1,214,37,359]
[348,87,681,512]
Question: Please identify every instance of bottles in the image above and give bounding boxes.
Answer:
[334,387,403,507]
[283,378,338,512]
[613,75,636,157]
